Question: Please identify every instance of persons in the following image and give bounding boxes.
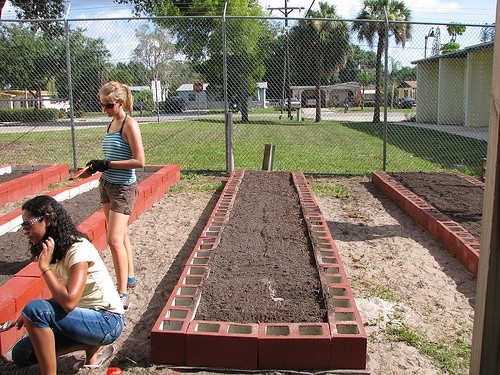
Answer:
[12,195,124,375]
[80,81,145,309]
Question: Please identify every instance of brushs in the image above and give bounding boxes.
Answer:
[0,321,16,332]
[69,165,90,180]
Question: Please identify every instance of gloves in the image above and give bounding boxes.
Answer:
[86,160,111,174]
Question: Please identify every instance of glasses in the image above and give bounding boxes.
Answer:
[21,216,46,230]
[100,100,120,108]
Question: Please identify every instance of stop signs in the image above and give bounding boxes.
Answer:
[193,83,203,93]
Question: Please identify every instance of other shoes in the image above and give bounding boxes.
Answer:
[119,293,128,305]
[127,277,137,287]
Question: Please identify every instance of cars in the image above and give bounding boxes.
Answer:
[285,98,301,110]
[399,97,416,108]
[165,97,186,112]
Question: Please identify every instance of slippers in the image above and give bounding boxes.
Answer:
[74,345,115,370]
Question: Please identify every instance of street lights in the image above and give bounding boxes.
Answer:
[424,27,436,57]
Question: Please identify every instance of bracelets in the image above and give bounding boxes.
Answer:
[42,269,52,275]
